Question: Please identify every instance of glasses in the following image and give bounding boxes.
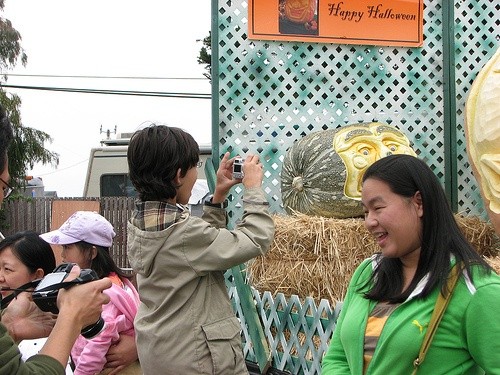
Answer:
[190,161,203,169]
[0,177,13,199]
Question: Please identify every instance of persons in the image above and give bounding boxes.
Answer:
[322,154,500,375]
[39,211,141,375]
[0,230,139,375]
[0,106,112,375]
[125,125,275,375]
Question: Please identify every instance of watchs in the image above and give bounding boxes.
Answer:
[204,195,229,208]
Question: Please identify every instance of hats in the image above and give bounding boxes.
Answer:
[39,211,117,247]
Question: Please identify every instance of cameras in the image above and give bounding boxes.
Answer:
[32,262,107,341]
[233,159,244,179]
